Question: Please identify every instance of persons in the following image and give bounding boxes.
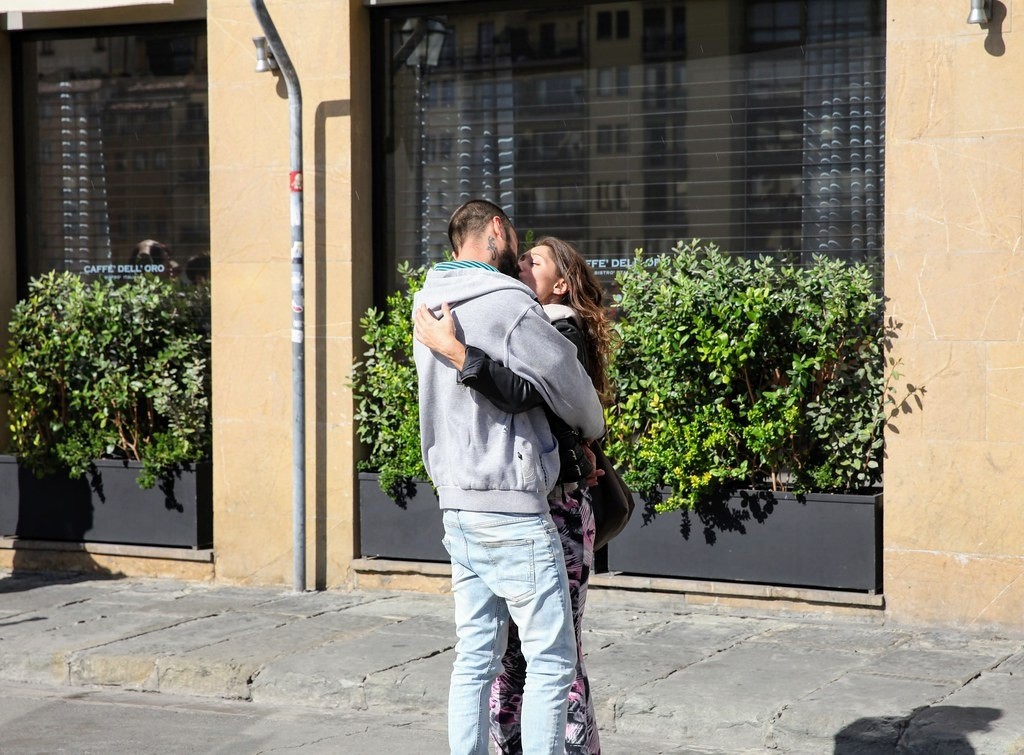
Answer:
[412,199,635,755]
[129,240,211,286]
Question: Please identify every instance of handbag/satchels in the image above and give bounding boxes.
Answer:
[589,441,634,574]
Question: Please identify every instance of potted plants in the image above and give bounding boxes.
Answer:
[341,236,902,592]
[0,262,212,550]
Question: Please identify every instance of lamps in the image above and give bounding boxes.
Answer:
[253,35,279,73]
[966,0,993,23]
